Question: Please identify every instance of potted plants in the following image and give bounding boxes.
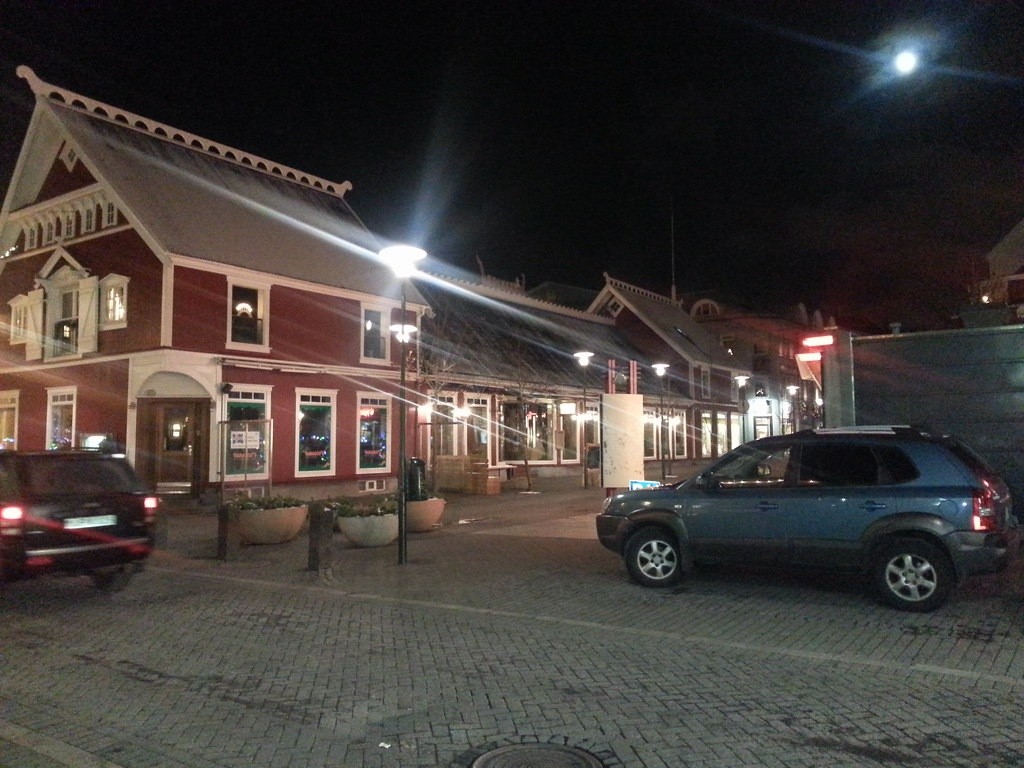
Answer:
[399,460,445,533]
[236,494,309,544]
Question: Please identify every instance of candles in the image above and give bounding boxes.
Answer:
[335,497,400,548]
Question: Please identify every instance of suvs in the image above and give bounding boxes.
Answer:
[596,424,1019,613]
[0,446,159,593]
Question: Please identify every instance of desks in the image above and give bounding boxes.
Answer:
[487,465,517,478]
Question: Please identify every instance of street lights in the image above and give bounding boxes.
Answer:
[786,385,800,435]
[815,398,823,427]
[377,245,428,566]
[651,364,671,481]
[734,375,750,444]
[573,350,595,488]
